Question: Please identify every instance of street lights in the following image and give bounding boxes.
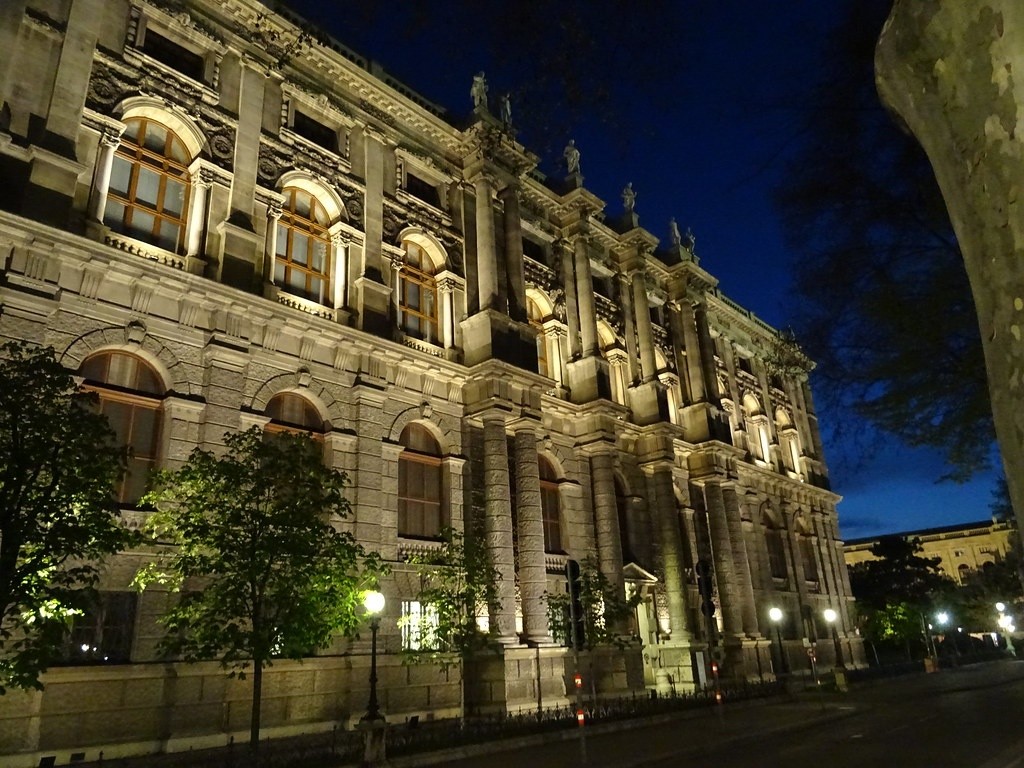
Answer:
[769,607,790,674]
[362,590,386,723]
[824,608,848,673]
[996,600,1016,658]
